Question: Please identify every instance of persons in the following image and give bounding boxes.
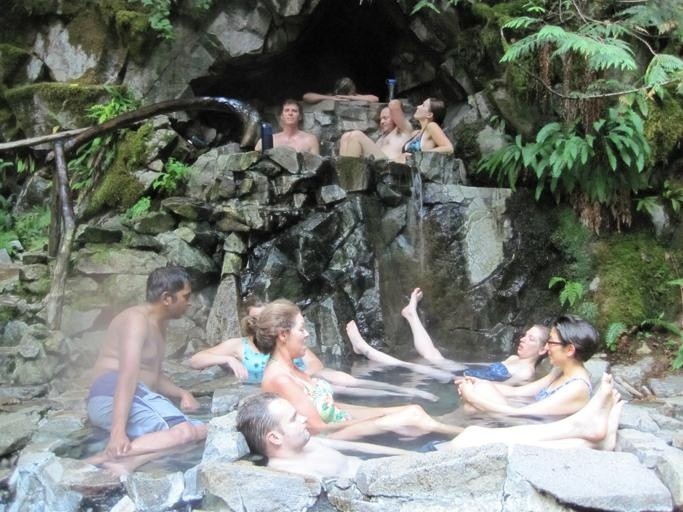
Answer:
[302,78,378,105]
[238,372,625,484]
[375,97,416,161]
[344,285,553,390]
[255,99,320,157]
[189,299,441,407]
[339,96,455,164]
[243,298,468,442]
[429,311,602,429]
[82,263,207,477]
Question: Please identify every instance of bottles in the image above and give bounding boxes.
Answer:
[261,121,273,153]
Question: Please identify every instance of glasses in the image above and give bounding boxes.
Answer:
[547,337,569,347]
